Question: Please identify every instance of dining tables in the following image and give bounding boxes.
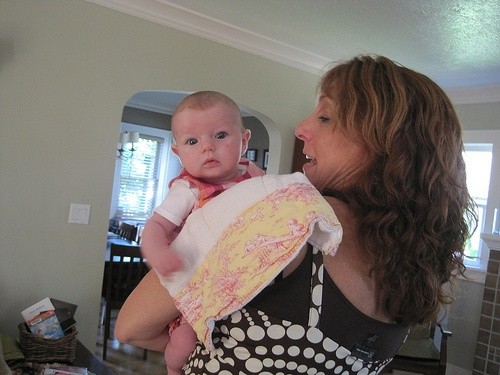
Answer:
[100,231,146,298]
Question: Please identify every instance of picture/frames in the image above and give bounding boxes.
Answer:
[262,149,269,170]
[245,148,258,162]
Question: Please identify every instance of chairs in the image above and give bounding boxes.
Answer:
[379,322,452,375]
[102,242,150,362]
[117,222,136,242]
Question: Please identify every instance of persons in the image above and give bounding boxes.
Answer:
[116,58,476,375]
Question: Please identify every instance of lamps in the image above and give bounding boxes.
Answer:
[117,128,139,161]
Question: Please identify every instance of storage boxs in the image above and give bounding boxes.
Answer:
[18,320,78,363]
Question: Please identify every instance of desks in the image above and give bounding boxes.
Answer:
[14,339,114,375]
